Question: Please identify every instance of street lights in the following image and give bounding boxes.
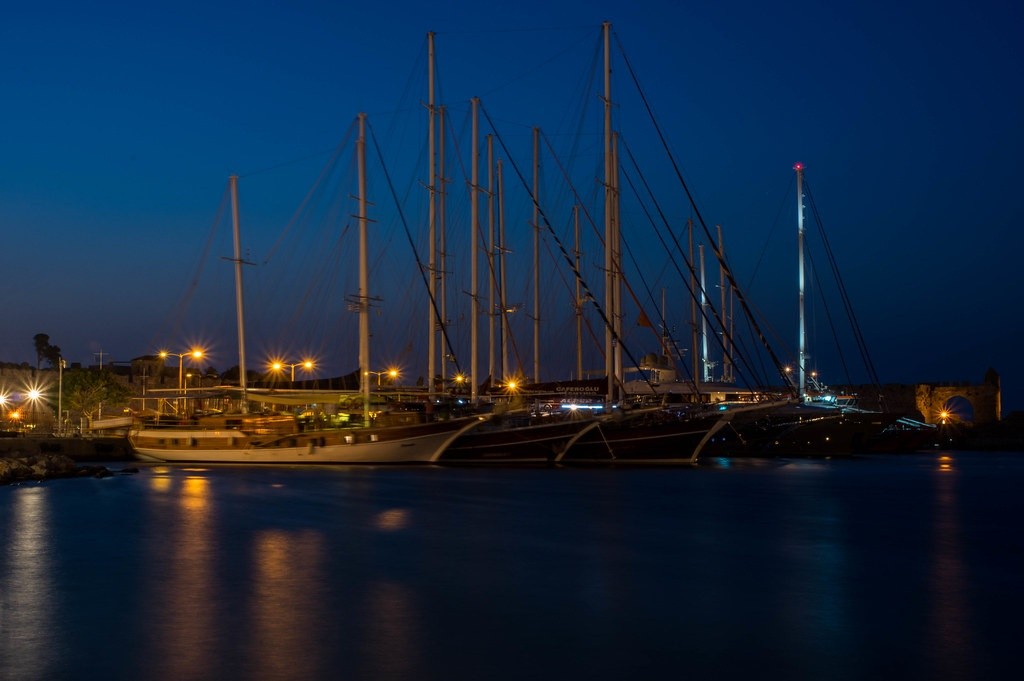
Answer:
[184,374,192,414]
[364,368,398,405]
[160,350,202,413]
[271,360,313,383]
[28,389,40,428]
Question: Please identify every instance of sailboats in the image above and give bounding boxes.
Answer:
[124,19,1021,469]
